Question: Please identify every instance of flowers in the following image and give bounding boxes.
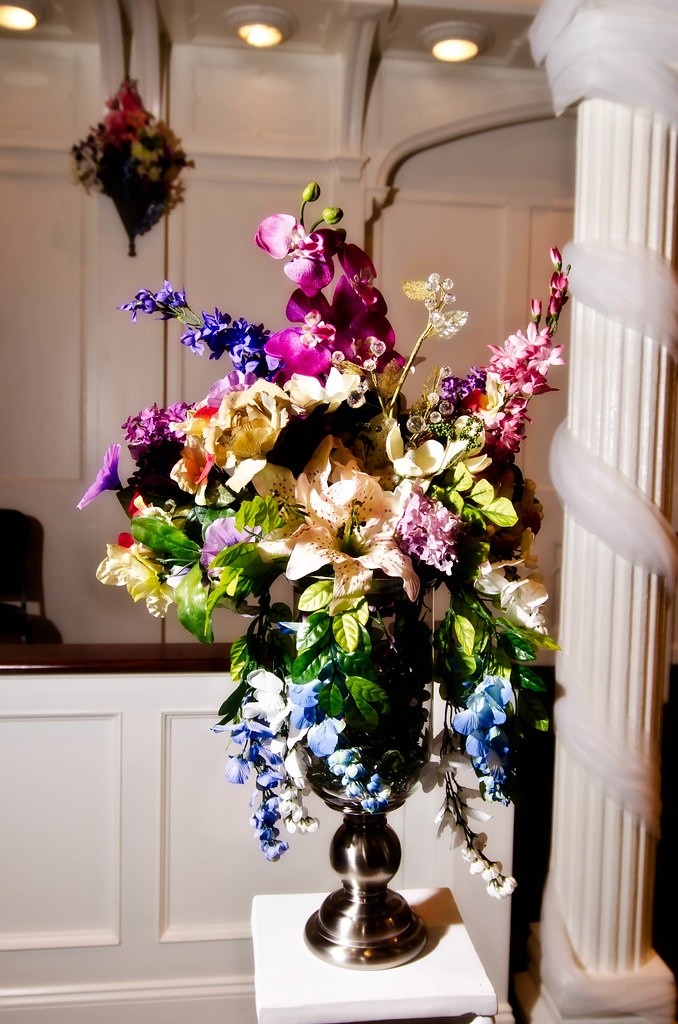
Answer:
[70,81,198,257]
[74,182,573,901]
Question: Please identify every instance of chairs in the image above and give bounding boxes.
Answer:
[0,507,62,643]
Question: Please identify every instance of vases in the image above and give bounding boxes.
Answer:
[305,766,427,971]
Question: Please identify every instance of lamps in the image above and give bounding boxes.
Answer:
[225,6,296,48]
[418,18,491,64]
[2,1,48,32]
